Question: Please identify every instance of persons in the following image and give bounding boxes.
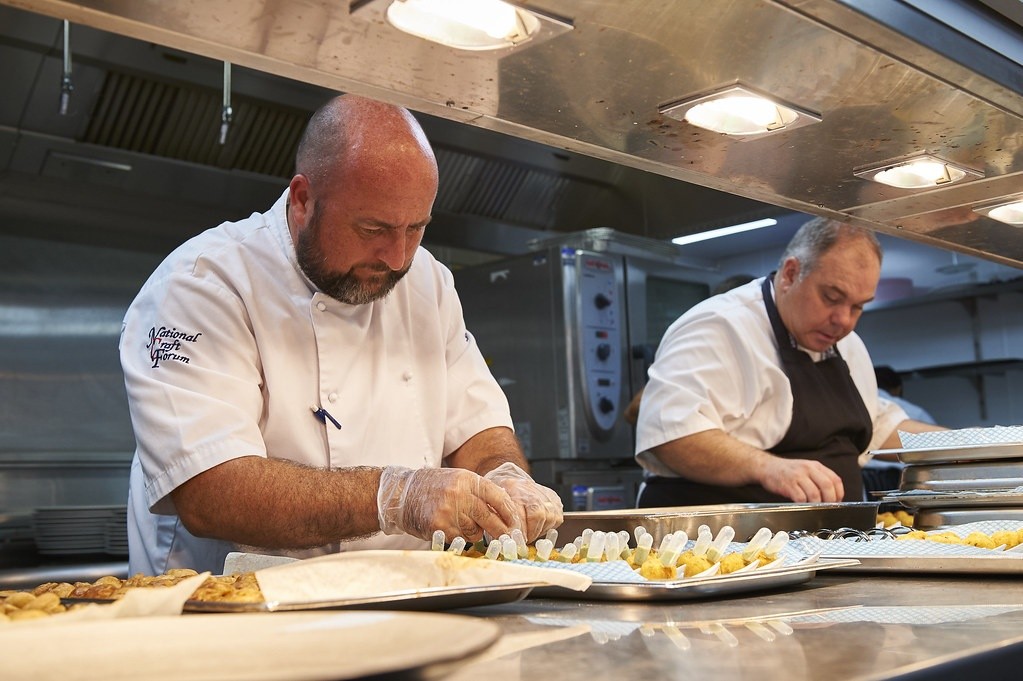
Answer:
[623,215,958,515]
[119,94,564,581]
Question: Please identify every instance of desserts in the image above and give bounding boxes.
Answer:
[876,510,915,527]
[897,526,1023,552]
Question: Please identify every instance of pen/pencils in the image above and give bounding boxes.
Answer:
[309,403,342,431]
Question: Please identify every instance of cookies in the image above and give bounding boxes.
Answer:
[0,568,263,628]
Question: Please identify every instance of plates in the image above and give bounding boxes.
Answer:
[31,506,129,554]
[0,611,501,681]
